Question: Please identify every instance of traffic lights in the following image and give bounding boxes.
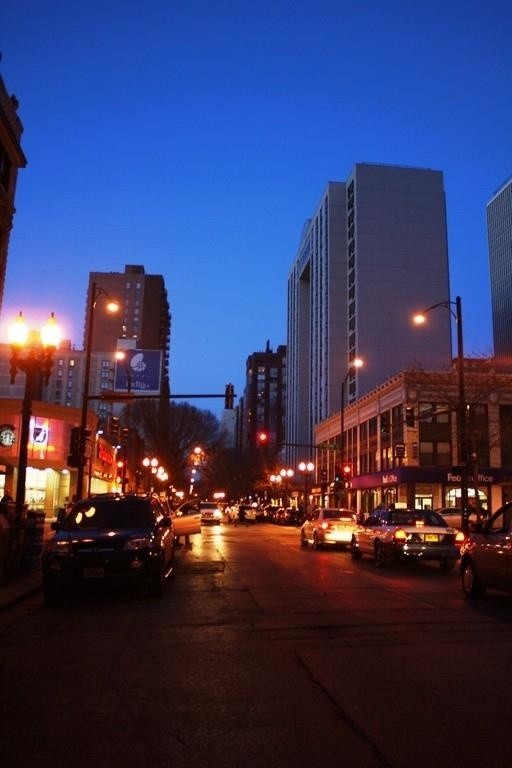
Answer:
[114,460,126,485]
[379,415,391,434]
[405,404,415,429]
[257,429,268,446]
[68,426,92,468]
[343,466,351,489]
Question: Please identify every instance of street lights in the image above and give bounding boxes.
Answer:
[414,297,469,539]
[12,311,61,505]
[74,282,120,497]
[143,455,170,495]
[341,358,365,506]
[270,461,314,523]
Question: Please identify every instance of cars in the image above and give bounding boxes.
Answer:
[42,494,301,595]
[301,501,512,600]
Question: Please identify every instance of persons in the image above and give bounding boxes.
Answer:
[297,505,304,524]
[233,507,249,528]
[174,498,199,545]
